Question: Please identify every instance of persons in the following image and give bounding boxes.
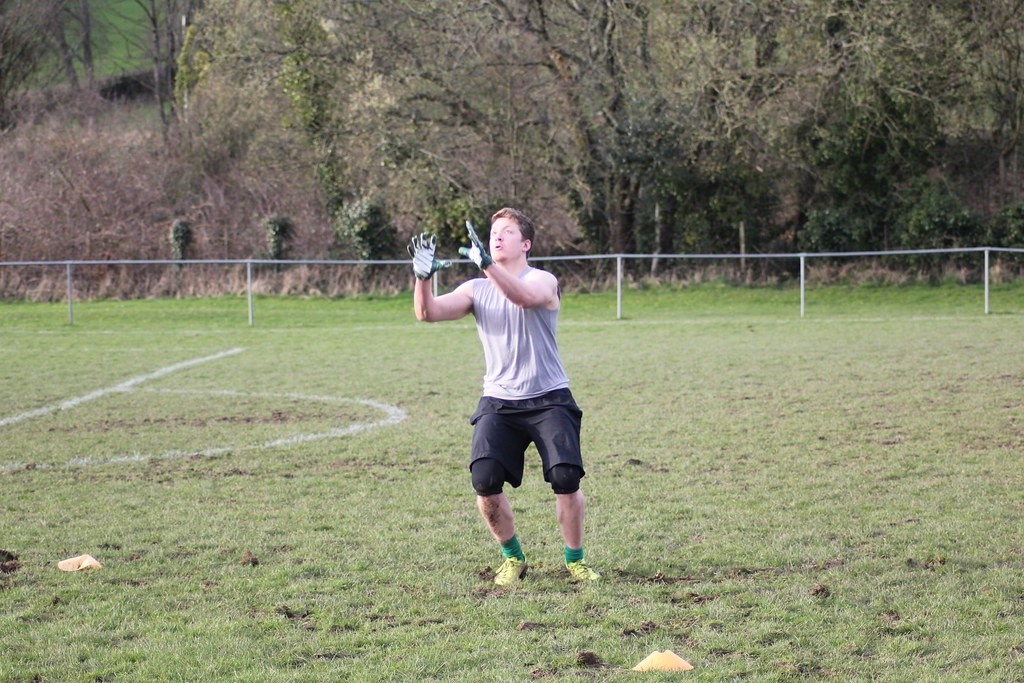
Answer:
[407,208,601,586]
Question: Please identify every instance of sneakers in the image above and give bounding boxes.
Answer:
[493,552,529,587]
[563,557,605,583]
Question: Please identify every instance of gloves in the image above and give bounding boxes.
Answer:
[457,220,492,272]
[405,232,453,280]
